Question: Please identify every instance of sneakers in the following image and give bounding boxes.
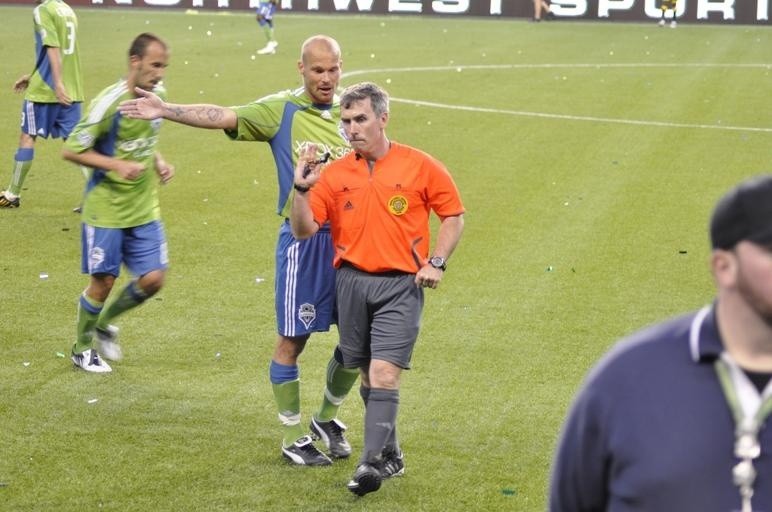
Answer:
[0,196,20,209]
[382,450,405,479]
[94,325,122,360]
[71,343,112,372]
[282,435,332,466]
[348,452,383,497]
[308,414,352,458]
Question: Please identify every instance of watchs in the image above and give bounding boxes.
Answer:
[428,255,448,271]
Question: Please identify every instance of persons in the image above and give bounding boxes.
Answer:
[535,0,554,22]
[60,35,176,373]
[658,0,677,29]
[543,174,772,512]
[115,34,362,466]
[292,82,465,497]
[1,0,95,212]
[256,1,278,55]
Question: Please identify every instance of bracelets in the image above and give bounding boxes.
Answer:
[294,183,310,193]
